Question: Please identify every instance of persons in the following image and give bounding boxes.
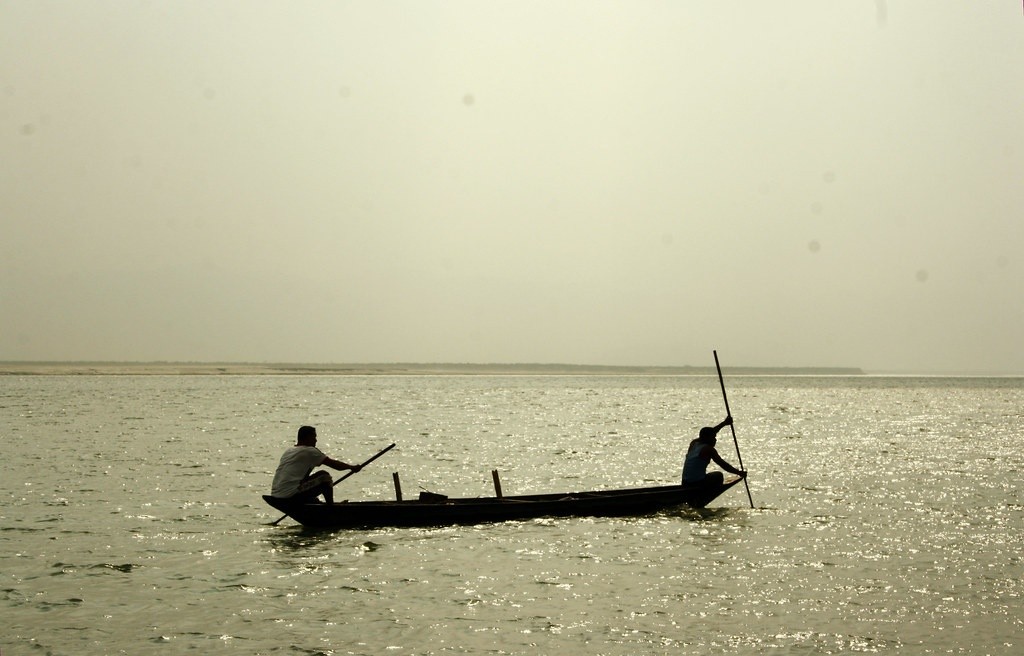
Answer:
[681,415,748,490]
[270,426,362,506]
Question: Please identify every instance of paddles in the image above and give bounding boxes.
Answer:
[260,443,397,526]
[713,349,755,508]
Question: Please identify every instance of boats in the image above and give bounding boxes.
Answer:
[261,475,744,531]
